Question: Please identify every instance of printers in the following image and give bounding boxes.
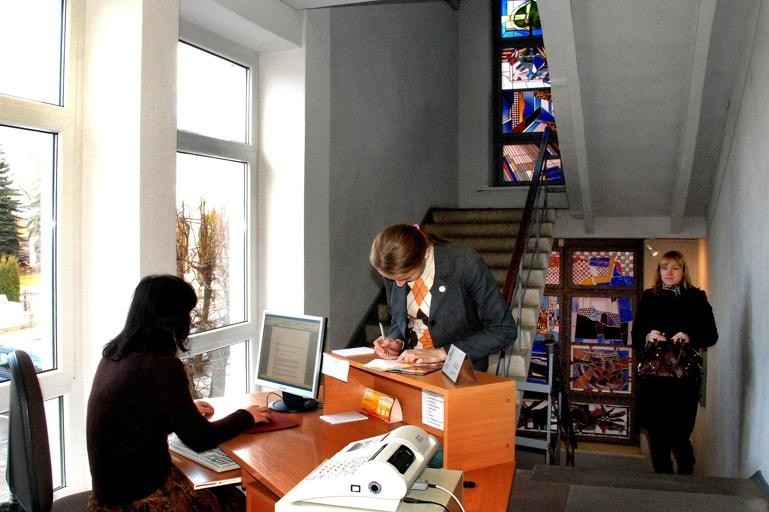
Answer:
[274,424,438,512]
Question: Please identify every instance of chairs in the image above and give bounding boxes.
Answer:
[5,350,93,511]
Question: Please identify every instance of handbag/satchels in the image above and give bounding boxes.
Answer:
[632,339,695,378]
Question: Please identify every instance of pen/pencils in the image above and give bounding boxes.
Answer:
[379,322,389,357]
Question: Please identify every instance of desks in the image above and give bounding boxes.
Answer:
[168,346,515,511]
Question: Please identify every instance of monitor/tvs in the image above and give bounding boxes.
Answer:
[254,310,328,413]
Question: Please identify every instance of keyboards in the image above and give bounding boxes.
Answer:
[167,432,241,473]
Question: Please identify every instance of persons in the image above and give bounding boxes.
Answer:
[86,275,273,512]
[369,223,517,372]
[631,251,718,475]
